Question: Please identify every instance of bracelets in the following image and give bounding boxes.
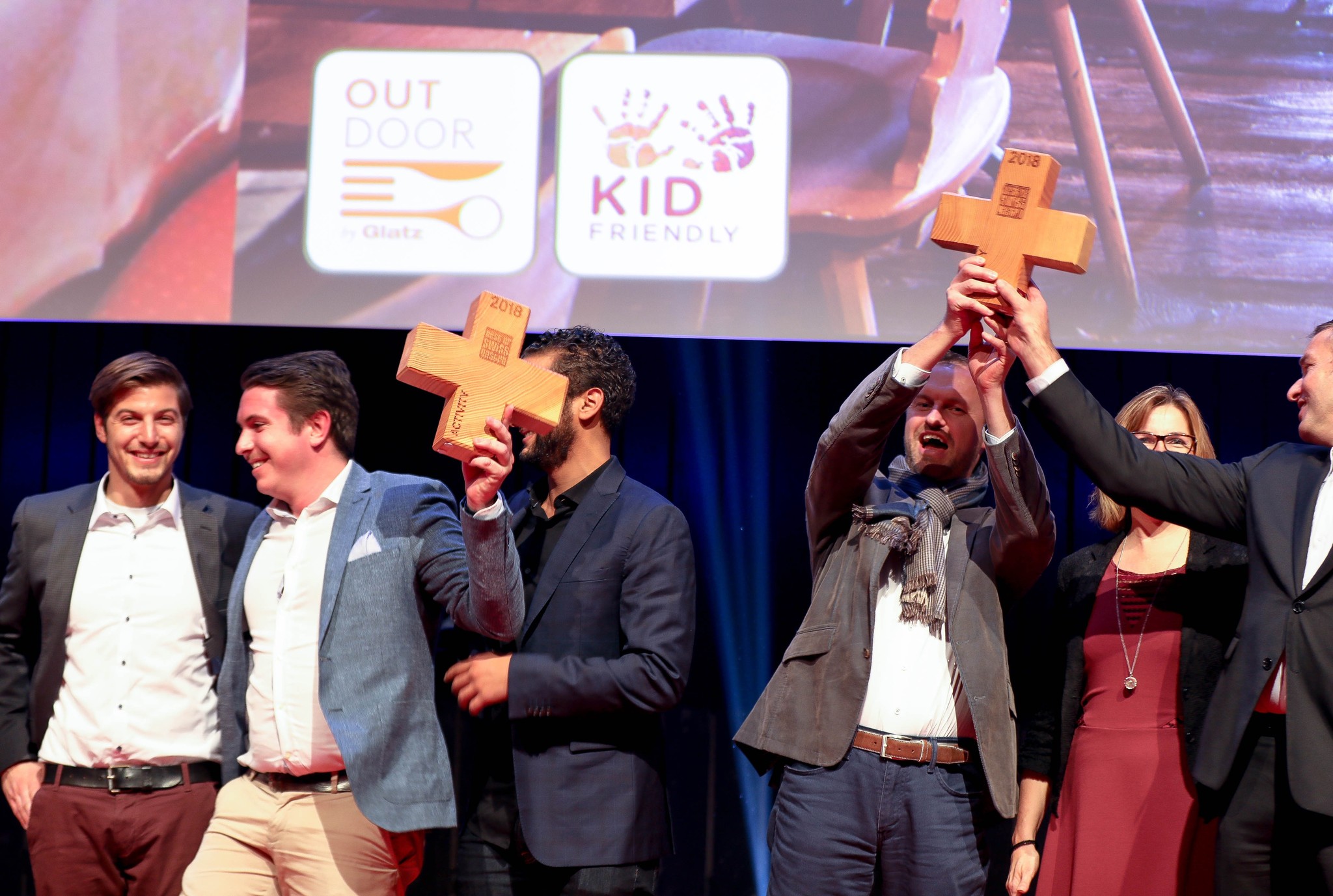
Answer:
[1011,840,1035,851]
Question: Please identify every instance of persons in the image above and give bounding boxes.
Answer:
[0,353,262,896]
[422,323,695,895]
[180,352,515,896]
[732,254,1333,896]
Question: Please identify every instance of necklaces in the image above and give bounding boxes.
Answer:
[1114,523,1192,691]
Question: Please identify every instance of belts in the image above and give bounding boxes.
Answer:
[245,767,351,792]
[40,762,217,793]
[850,729,970,765]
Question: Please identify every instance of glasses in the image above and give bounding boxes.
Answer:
[1130,432,1197,454]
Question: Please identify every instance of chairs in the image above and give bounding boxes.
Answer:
[637,2,1015,342]
